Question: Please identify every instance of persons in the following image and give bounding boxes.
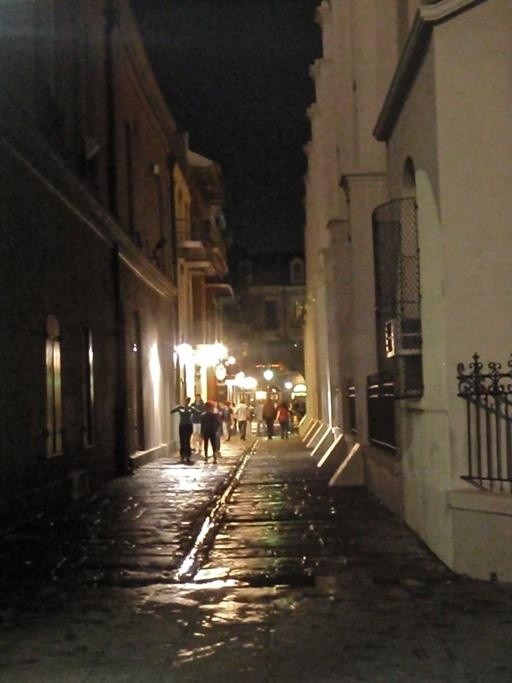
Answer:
[169,388,307,463]
